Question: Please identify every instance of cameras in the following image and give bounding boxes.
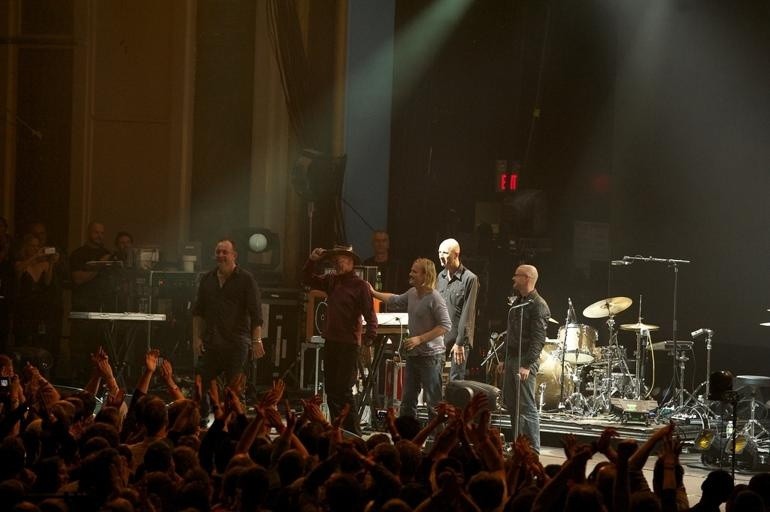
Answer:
[44,248,56,254]
[377,409,388,418]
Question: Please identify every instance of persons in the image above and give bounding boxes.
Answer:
[0,218,63,358]
[361,230,409,393]
[192,240,265,428]
[434,238,480,380]
[299,240,378,437]
[497,263,551,459]
[112,232,150,368]
[468,224,507,366]
[69,221,116,367]
[364,257,452,453]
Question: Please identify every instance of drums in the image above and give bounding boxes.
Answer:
[612,372,635,390]
[621,323,660,330]
[543,338,561,355]
[592,368,608,388]
[537,350,573,406]
[557,324,598,364]
[600,345,627,362]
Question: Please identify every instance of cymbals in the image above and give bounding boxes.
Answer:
[508,295,559,323]
[582,296,633,319]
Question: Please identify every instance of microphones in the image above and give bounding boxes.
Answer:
[611,261,628,265]
[510,299,534,309]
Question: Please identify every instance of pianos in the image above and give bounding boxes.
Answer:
[68,311,167,322]
[361,313,409,335]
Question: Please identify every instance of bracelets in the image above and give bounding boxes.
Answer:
[251,337,262,344]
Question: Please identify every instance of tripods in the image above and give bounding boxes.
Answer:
[545,317,719,419]
[505,313,539,455]
[738,399,770,449]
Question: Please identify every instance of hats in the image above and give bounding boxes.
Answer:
[325,241,361,266]
